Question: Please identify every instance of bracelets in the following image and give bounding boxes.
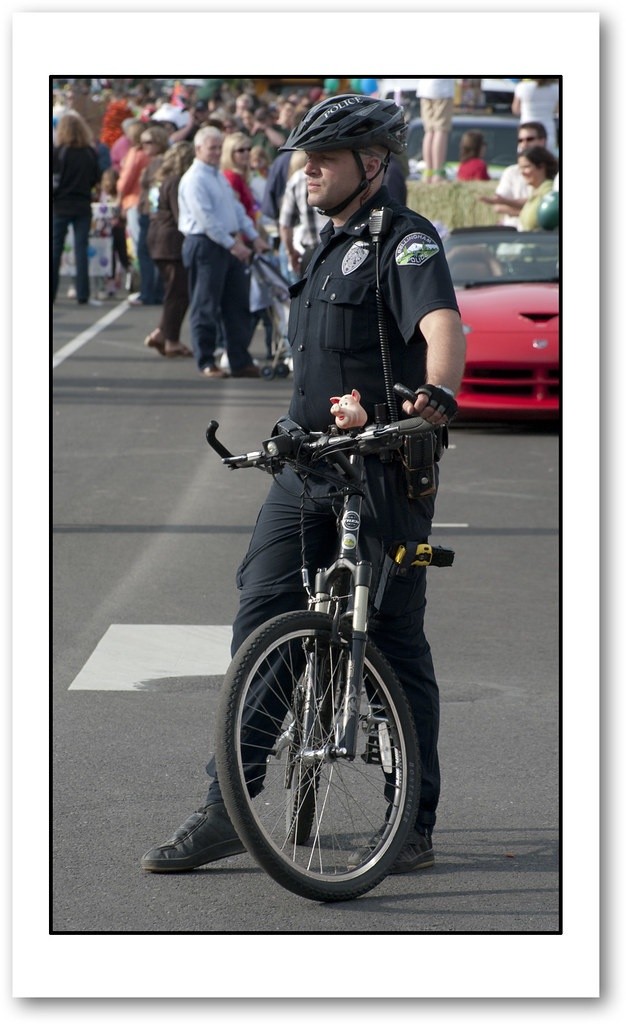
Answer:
[436,385,454,397]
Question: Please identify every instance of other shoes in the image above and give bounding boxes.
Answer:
[212,352,258,373]
[233,364,262,378]
[165,343,193,358]
[200,365,228,379]
[143,336,165,356]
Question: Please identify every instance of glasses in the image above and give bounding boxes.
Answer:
[517,136,544,143]
[233,147,252,153]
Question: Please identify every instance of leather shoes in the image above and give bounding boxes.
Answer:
[140,802,249,873]
[346,819,436,876]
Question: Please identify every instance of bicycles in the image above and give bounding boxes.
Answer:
[207,381,456,905]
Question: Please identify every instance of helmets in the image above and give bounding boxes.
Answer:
[277,94,407,155]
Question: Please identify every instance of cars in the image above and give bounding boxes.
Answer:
[375,78,516,116]
[441,227,560,429]
[402,115,521,183]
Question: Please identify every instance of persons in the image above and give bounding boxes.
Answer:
[479,122,559,232]
[142,94,465,870]
[512,79,558,152]
[457,130,492,180]
[417,79,457,184]
[53,79,327,377]
